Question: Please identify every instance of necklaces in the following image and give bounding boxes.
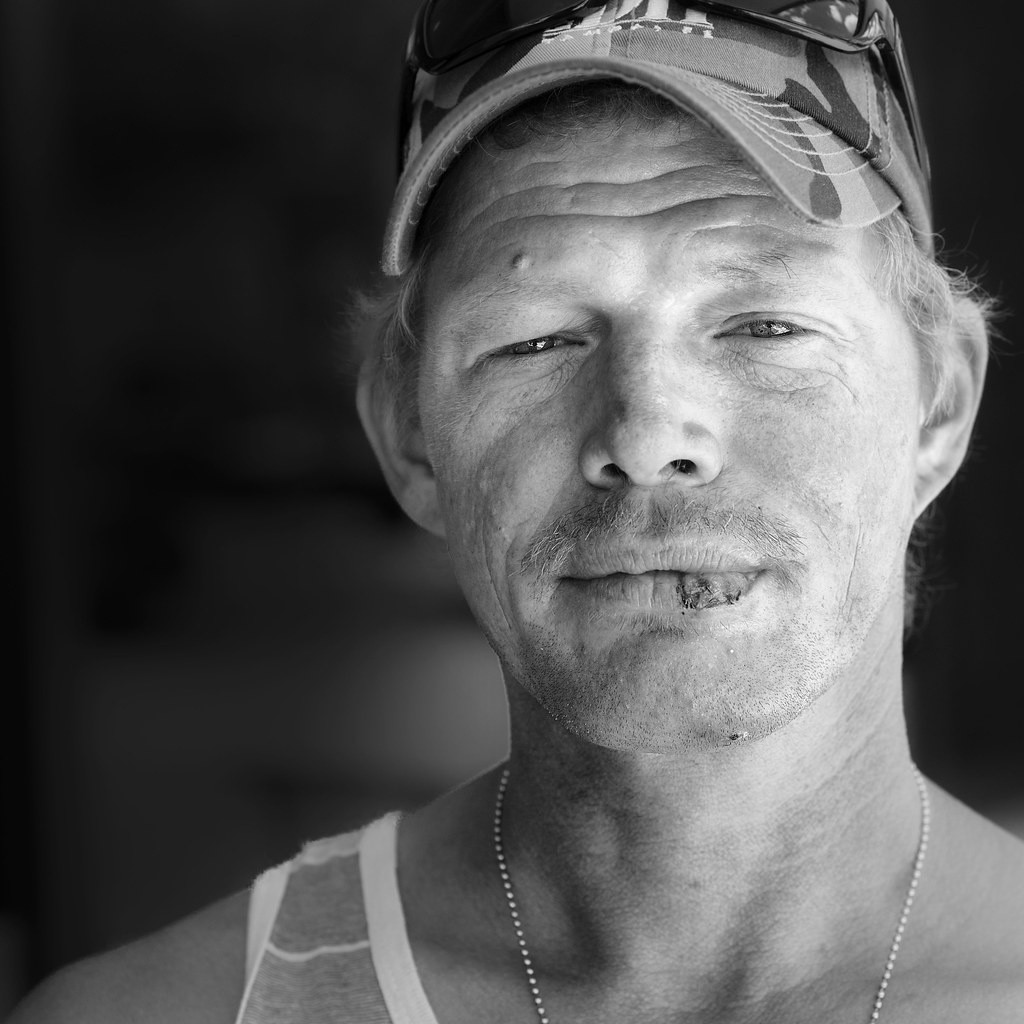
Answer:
[494,759,930,1024]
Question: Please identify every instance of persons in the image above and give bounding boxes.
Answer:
[15,0,1022,1024]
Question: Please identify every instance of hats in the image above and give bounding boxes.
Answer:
[379,0,936,277]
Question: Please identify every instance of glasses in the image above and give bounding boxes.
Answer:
[392,0,925,189]
